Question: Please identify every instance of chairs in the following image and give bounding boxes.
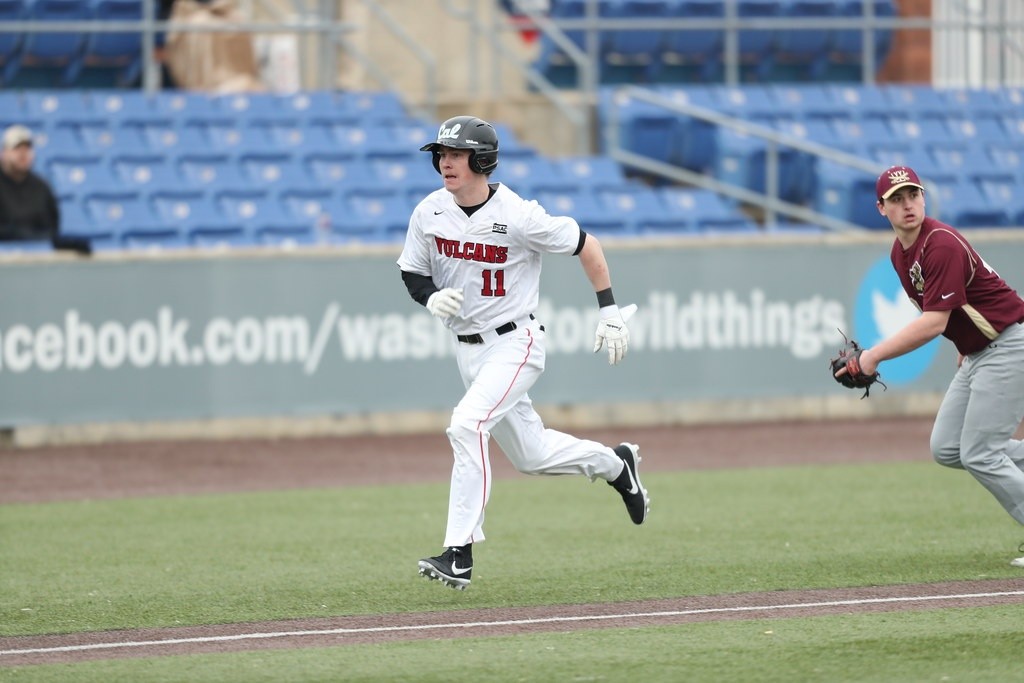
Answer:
[0,0,1024,277]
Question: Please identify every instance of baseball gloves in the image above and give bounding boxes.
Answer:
[829,340,878,389]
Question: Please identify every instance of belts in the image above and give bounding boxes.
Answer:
[457,313,535,344]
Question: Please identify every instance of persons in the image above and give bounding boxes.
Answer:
[0,123,59,241]
[830,166,1024,569]
[396,115,652,591]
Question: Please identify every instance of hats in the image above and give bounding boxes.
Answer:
[876,166,924,200]
[4,124,34,149]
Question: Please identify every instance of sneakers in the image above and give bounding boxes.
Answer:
[418,547,473,591]
[607,442,650,525]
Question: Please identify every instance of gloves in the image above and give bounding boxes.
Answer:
[593,303,638,365]
[426,287,463,319]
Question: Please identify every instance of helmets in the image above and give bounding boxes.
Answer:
[419,115,499,174]
